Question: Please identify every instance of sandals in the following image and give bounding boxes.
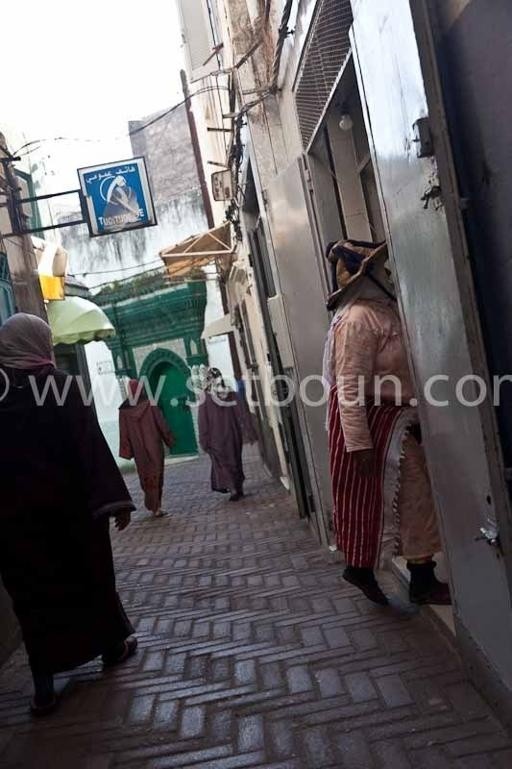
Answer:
[30,692,61,716]
[102,635,137,667]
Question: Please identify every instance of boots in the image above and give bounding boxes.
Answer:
[325,240,389,311]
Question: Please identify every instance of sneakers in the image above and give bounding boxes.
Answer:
[406,561,450,605]
[229,491,243,502]
[342,565,390,606]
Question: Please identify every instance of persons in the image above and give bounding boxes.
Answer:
[0,312,137,716]
[119,379,178,521]
[321,240,452,605]
[196,368,261,505]
